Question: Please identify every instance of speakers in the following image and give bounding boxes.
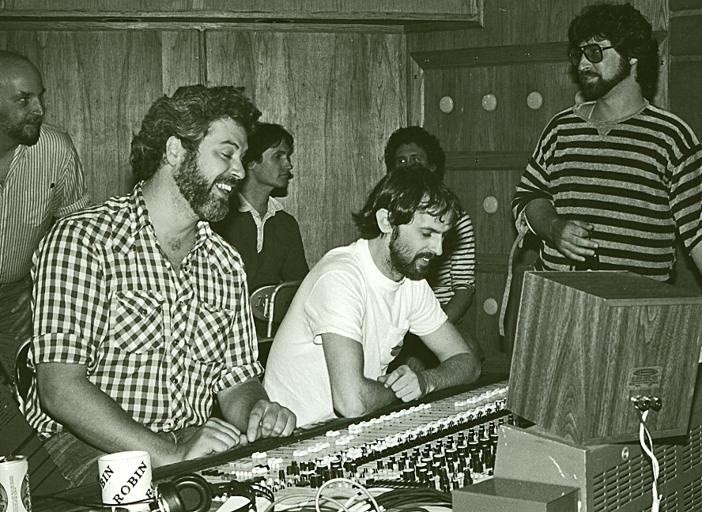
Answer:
[504,268,701,447]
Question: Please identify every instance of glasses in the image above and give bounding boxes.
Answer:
[568,42,617,67]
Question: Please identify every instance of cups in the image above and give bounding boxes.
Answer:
[97,449,153,511]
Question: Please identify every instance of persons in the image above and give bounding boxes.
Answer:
[210,121,309,340]
[1,50,90,498]
[510,3,702,284]
[262,164,481,429]
[385,126,476,326]
[16,83,296,488]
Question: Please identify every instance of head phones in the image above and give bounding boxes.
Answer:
[154,473,278,511]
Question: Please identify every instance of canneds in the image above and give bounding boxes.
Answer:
[0,455,32,512]
